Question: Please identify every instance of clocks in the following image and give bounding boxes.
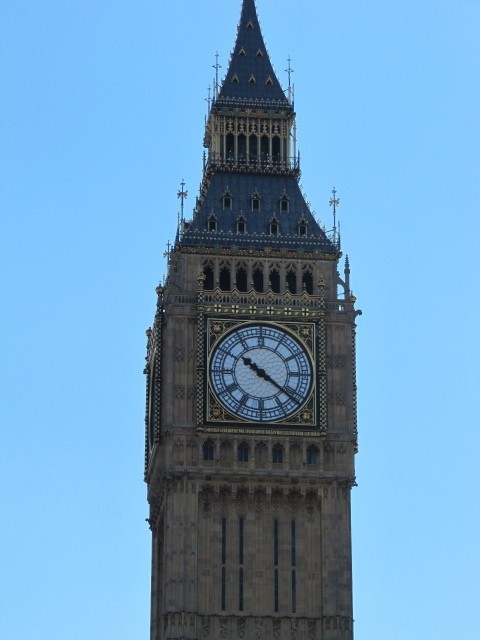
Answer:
[206,317,316,420]
